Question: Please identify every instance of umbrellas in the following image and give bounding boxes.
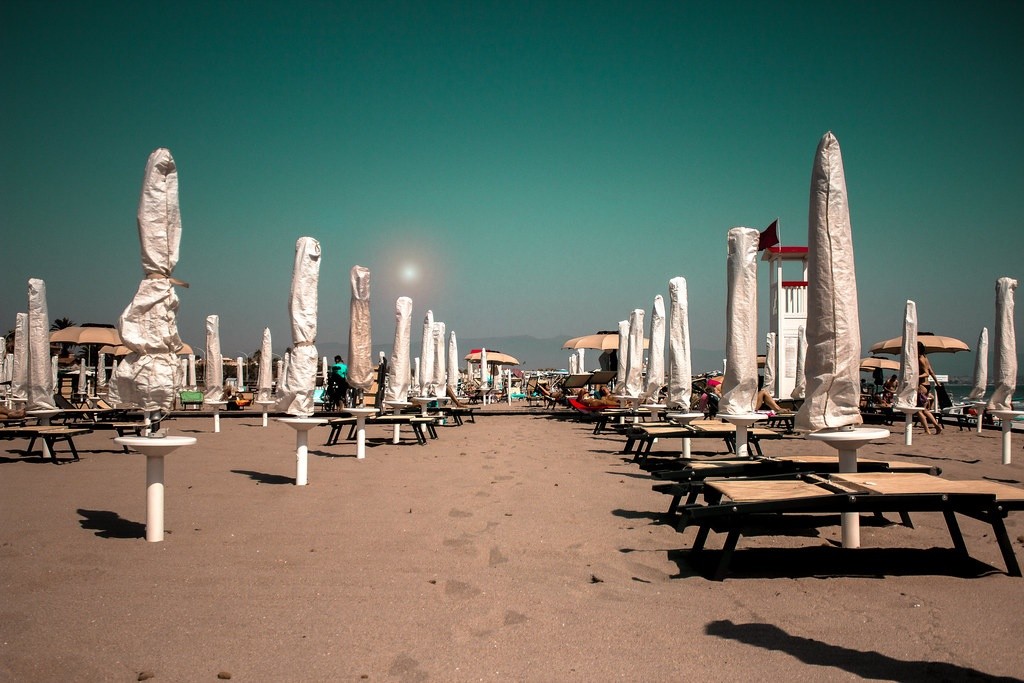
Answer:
[561,334,651,390]
[47,326,194,368]
[463,352,520,387]
[859,335,972,372]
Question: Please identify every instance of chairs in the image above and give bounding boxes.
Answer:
[0,382,1024,583]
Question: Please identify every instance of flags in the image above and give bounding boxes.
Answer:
[757,219,779,251]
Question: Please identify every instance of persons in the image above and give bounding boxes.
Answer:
[860,342,943,436]
[536,382,795,413]
[0,404,27,418]
[327,356,348,409]
